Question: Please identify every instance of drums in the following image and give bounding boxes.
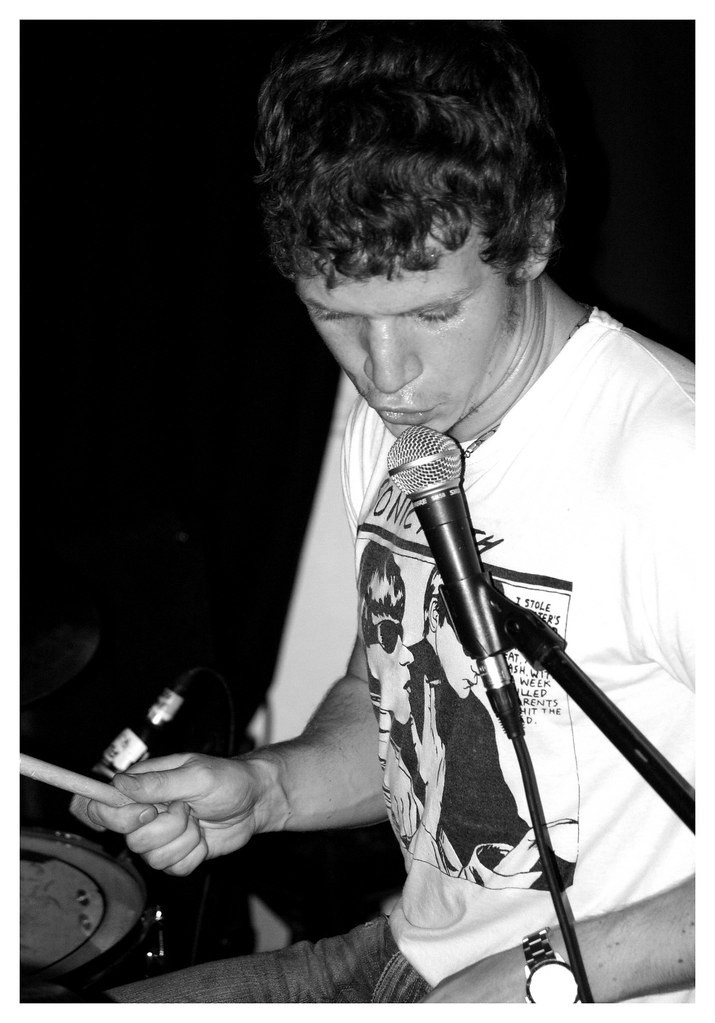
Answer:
[19,825,169,984]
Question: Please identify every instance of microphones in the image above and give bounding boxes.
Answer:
[69,663,203,831]
[386,422,524,741]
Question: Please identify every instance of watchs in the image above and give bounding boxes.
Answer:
[522,927,581,1004]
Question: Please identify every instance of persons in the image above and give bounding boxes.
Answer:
[86,20,695,1003]
